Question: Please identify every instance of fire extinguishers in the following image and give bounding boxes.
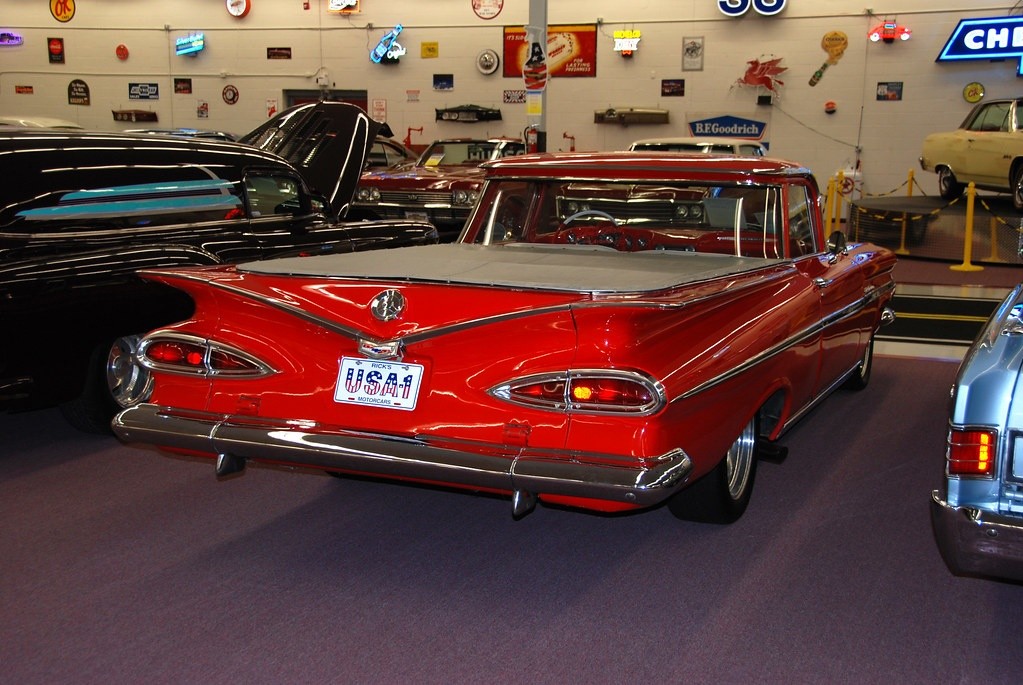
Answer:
[524,124,540,154]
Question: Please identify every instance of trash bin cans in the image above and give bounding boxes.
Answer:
[831,171,862,222]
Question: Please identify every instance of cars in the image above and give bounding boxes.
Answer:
[0,102,438,435]
[356,136,525,236]
[928,286,1023,576]
[555,138,772,226]
[97,149,892,524]
[918,97,1023,212]
